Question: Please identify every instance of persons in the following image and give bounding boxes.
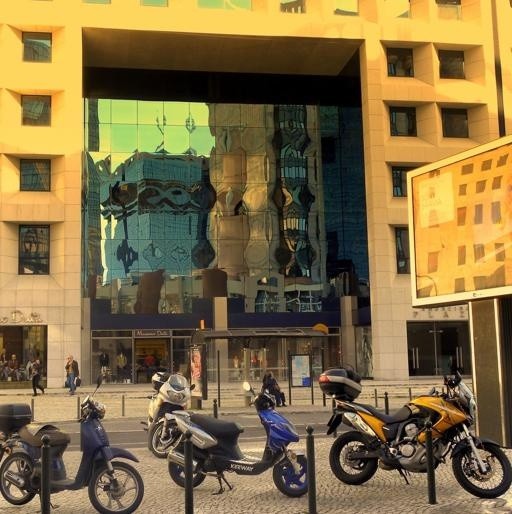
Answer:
[116,352,128,381]
[0,348,8,381]
[29,358,44,396]
[64,355,80,394]
[8,354,21,382]
[259,372,286,407]
[99,350,109,367]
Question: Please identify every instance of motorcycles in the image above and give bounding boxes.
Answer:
[0,371,147,514]
[317,365,512,498]
[165,381,309,498]
[139,368,196,460]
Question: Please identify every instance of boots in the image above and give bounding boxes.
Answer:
[32,388,44,396]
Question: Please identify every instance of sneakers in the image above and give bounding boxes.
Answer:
[277,404,287,407]
[69,387,76,395]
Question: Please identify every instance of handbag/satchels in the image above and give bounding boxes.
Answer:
[76,376,81,386]
